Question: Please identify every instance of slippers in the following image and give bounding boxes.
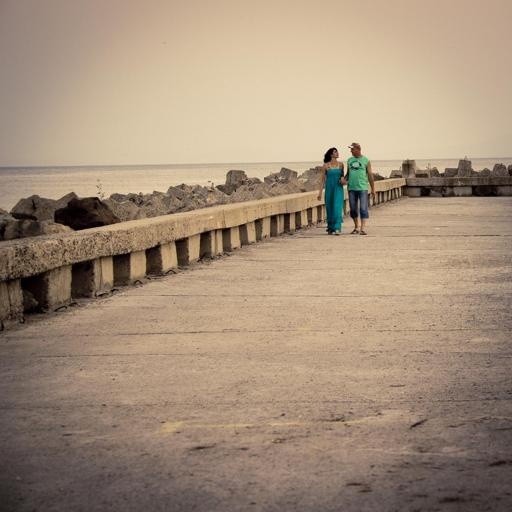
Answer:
[351,229,367,235]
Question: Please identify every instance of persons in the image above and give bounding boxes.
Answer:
[317,148,344,235]
[342,143,375,235]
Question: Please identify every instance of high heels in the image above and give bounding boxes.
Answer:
[326,228,342,235]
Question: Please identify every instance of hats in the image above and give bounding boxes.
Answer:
[348,143,360,149]
